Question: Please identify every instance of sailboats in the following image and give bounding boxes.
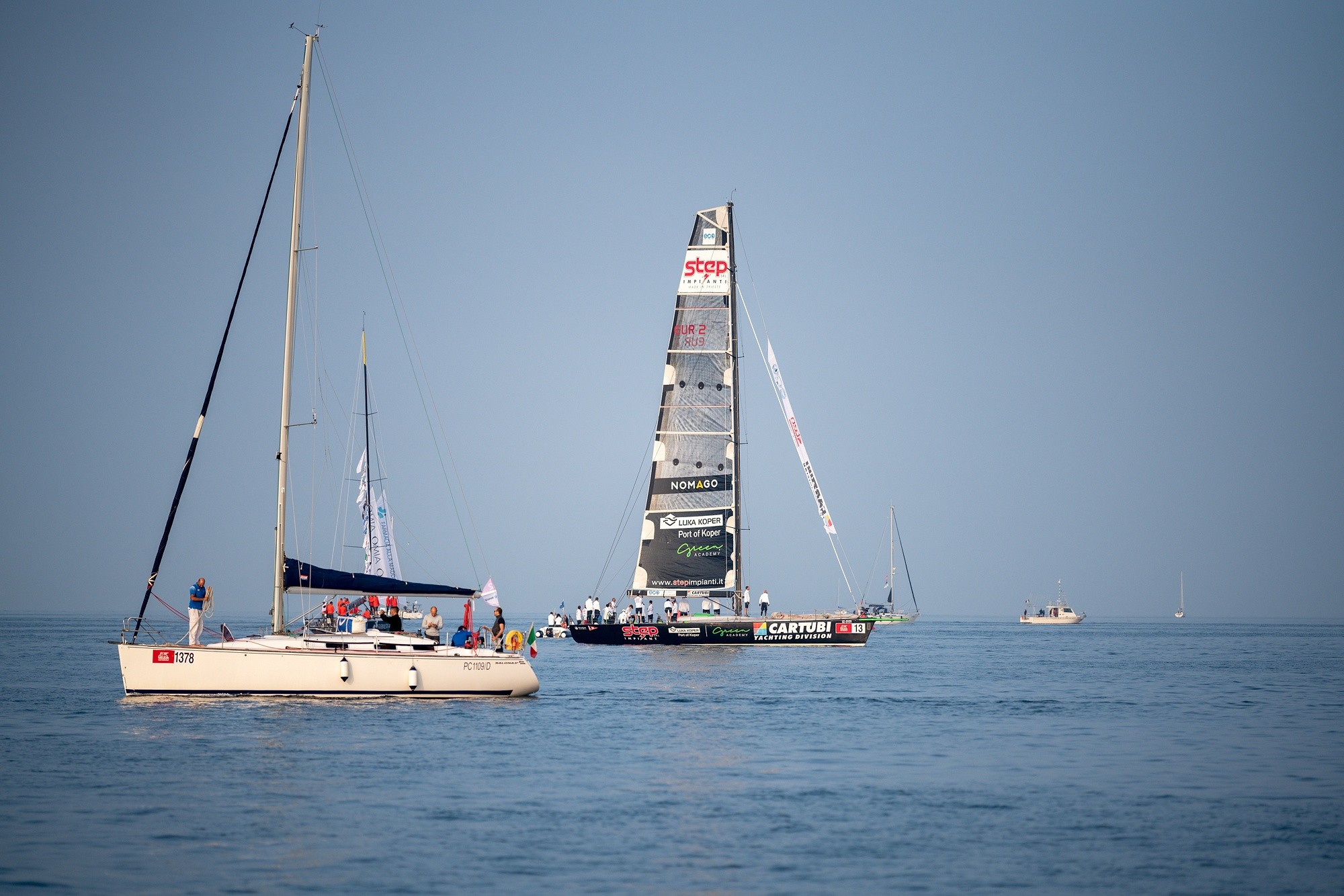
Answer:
[1174,569,1186,618]
[852,506,921,624]
[570,190,879,645]
[114,20,539,700]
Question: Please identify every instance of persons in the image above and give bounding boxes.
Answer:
[451,626,473,647]
[337,597,371,619]
[188,577,210,648]
[368,595,380,615]
[386,596,398,616]
[380,606,402,634]
[1024,608,1058,620]
[576,593,654,624]
[325,602,335,627]
[548,612,569,627]
[422,607,443,644]
[759,590,770,619]
[702,596,721,616]
[403,605,418,612]
[663,597,690,625]
[744,586,750,617]
[483,607,505,652]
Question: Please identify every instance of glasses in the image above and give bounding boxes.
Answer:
[493,610,498,613]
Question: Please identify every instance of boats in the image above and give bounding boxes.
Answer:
[535,626,571,638]
[1019,579,1088,624]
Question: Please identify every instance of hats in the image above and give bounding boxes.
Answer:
[622,609,626,612]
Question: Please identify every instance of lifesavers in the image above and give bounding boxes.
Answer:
[505,630,523,650]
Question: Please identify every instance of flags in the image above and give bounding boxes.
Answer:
[356,447,409,609]
[1025,599,1030,603]
[883,575,889,589]
[527,623,538,659]
[1083,608,1085,615]
[560,600,565,609]
